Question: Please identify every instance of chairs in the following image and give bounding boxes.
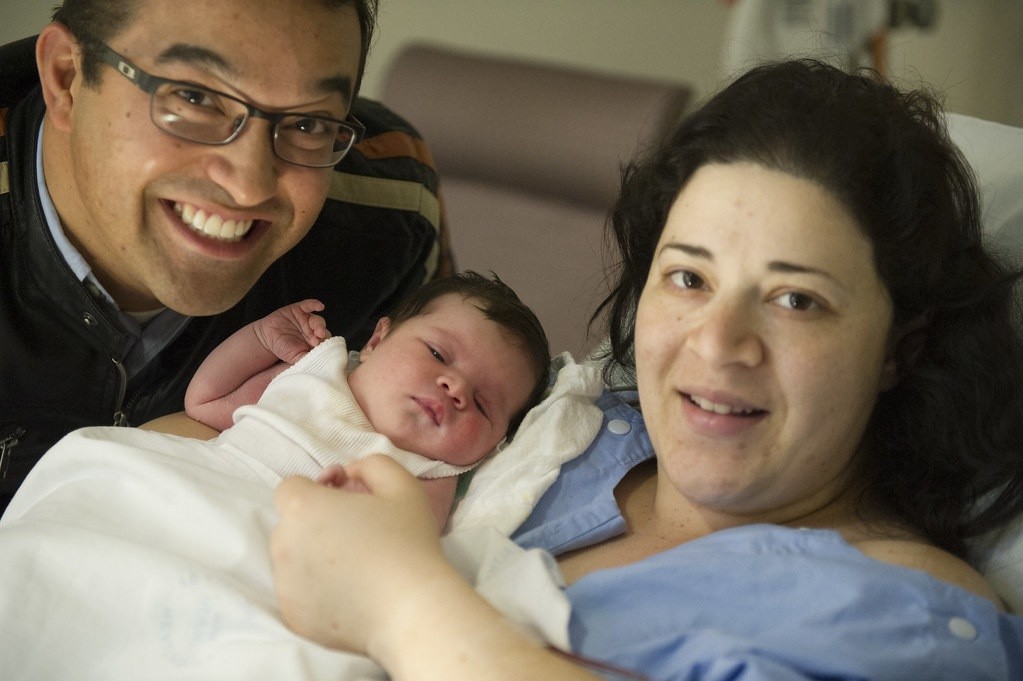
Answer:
[377,41,694,380]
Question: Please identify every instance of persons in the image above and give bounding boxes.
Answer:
[0,1,454,522]
[0,269,551,681]
[269,55,1023,681]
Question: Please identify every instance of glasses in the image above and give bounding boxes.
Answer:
[51,10,366,169]
